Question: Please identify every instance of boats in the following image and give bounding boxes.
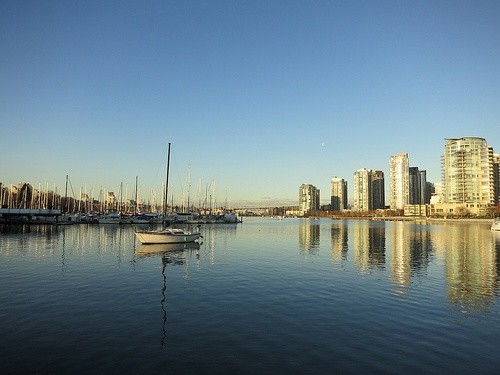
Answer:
[0,207,243,224]
[134,220,203,244]
[490,217,500,231]
